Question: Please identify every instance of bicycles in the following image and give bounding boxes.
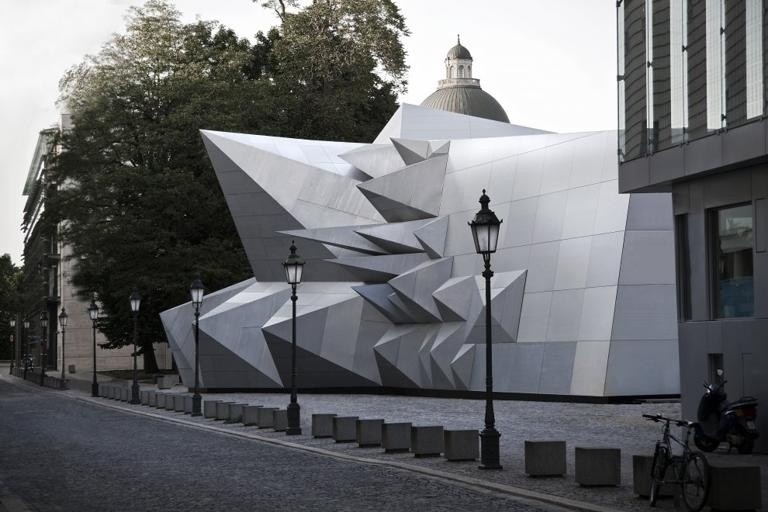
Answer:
[642,412,711,512]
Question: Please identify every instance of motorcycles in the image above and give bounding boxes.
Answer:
[694,380,760,454]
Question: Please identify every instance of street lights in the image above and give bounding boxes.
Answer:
[188,275,206,417]
[468,189,503,469]
[129,286,142,405]
[89,297,99,396]
[281,239,306,435]
[9,306,68,390]
